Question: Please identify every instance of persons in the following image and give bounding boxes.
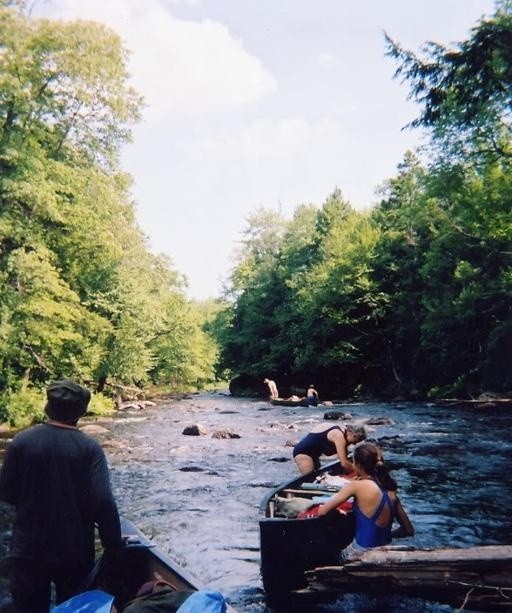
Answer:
[317,445,415,564]
[0,380,121,612]
[293,424,366,476]
[263,378,278,399]
[307,384,319,407]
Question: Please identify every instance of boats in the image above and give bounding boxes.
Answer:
[54,509,241,613]
[269,395,317,407]
[257,438,385,573]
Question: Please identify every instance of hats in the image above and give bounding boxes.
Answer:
[44,380,91,420]
